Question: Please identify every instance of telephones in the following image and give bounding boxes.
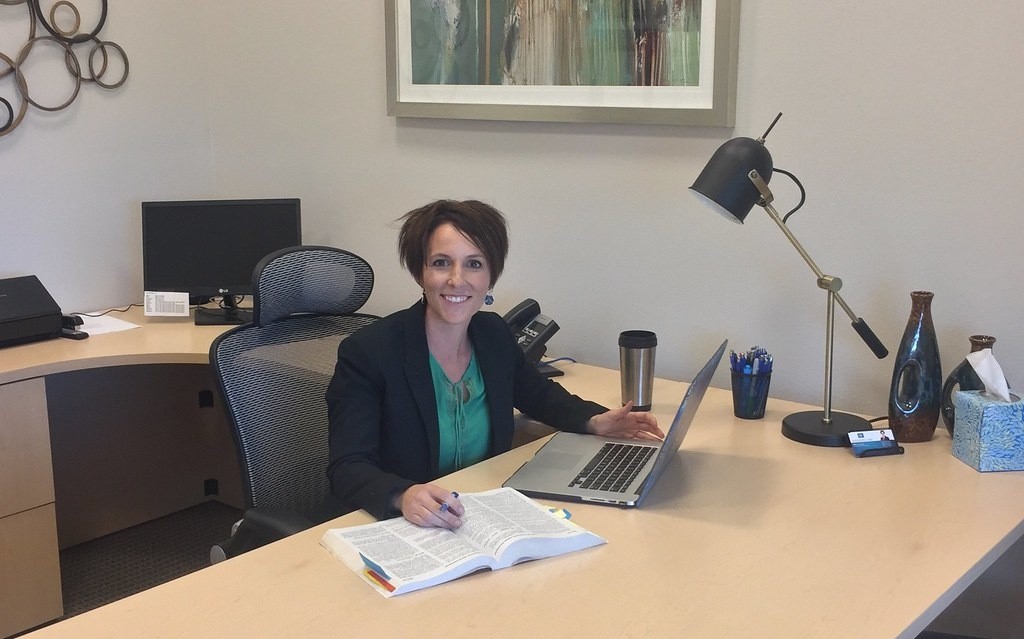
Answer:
[502,297,566,377]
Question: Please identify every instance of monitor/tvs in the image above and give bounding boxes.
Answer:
[142,199,302,325]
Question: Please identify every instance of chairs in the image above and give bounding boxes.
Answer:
[208,246,385,564]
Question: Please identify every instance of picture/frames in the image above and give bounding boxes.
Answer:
[384,0,740,128]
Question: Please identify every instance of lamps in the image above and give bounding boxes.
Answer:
[687,114,890,448]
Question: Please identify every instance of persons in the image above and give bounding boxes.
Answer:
[325,200,665,529]
[880,431,889,441]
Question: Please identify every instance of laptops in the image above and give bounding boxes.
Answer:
[501,339,729,507]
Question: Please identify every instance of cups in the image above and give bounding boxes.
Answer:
[618,330,657,412]
[729,368,772,419]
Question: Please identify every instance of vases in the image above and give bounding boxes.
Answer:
[888,291,942,443]
[941,334,1011,439]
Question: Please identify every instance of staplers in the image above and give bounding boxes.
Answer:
[62,314,89,340]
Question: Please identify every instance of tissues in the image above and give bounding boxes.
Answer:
[949,347,1023,472]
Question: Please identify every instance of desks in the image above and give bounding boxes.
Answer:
[0,300,1024,639]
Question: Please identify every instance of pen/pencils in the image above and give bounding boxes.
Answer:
[727,343,773,419]
[438,491,460,513]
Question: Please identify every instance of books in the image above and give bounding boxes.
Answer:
[320,487,607,598]
